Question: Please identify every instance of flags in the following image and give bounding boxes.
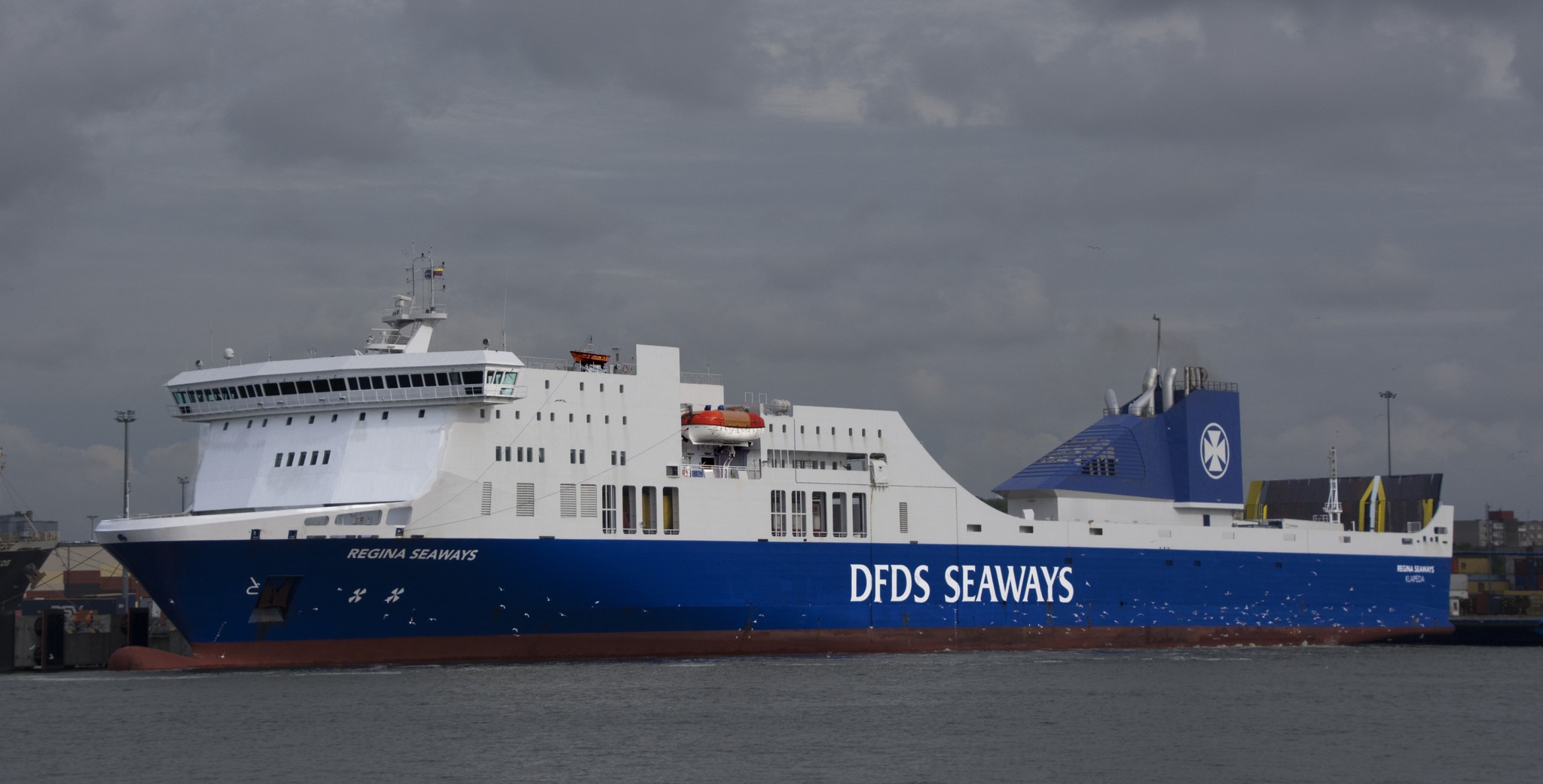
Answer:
[433,268,443,276]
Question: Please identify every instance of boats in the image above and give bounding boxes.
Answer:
[569,349,609,365]
[92,253,1468,672]
[682,411,766,446]
